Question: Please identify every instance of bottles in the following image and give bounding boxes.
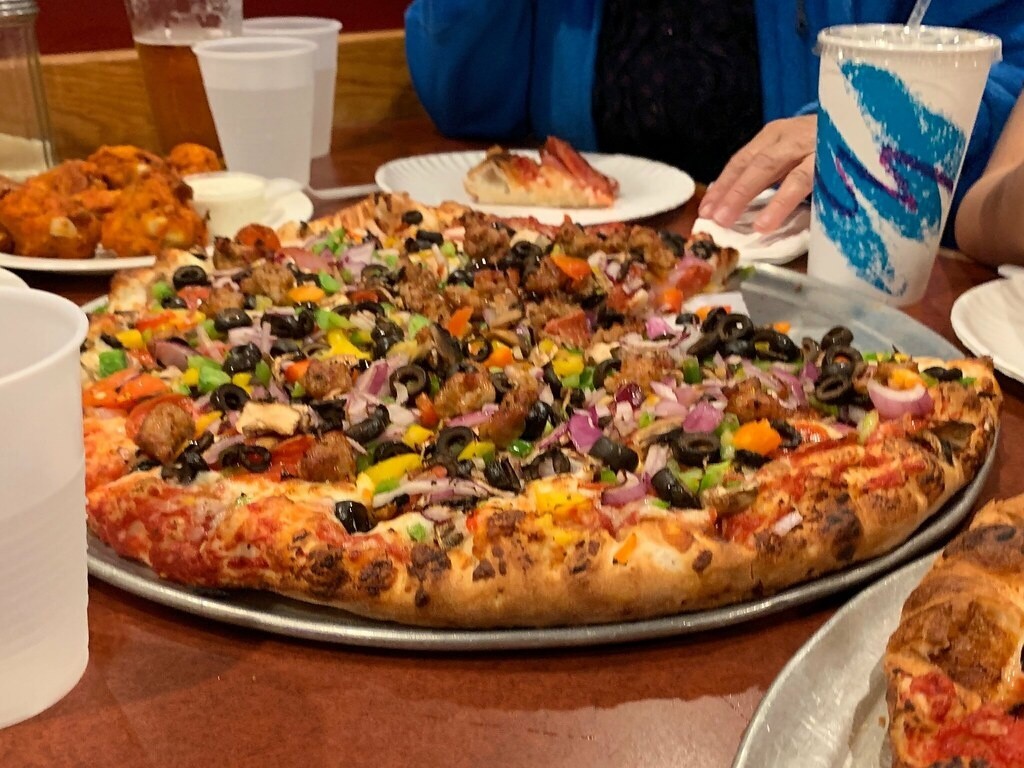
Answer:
[1,1,59,182]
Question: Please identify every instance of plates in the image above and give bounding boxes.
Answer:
[732,547,953,768]
[950,276,1024,383]
[374,150,695,227]
[1,171,315,270]
[84,261,999,648]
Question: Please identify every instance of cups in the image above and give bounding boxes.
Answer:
[192,37,317,187]
[809,24,1003,307]
[243,16,343,157]
[122,0,244,158]
[0,270,88,730]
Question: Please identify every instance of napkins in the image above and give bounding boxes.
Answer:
[691,182,812,266]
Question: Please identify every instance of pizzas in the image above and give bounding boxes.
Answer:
[466,136,619,207]
[881,492,1024,768]
[80,189,1001,631]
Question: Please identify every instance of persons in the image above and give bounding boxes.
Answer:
[956,89,1024,266]
[405,0,1024,254]
